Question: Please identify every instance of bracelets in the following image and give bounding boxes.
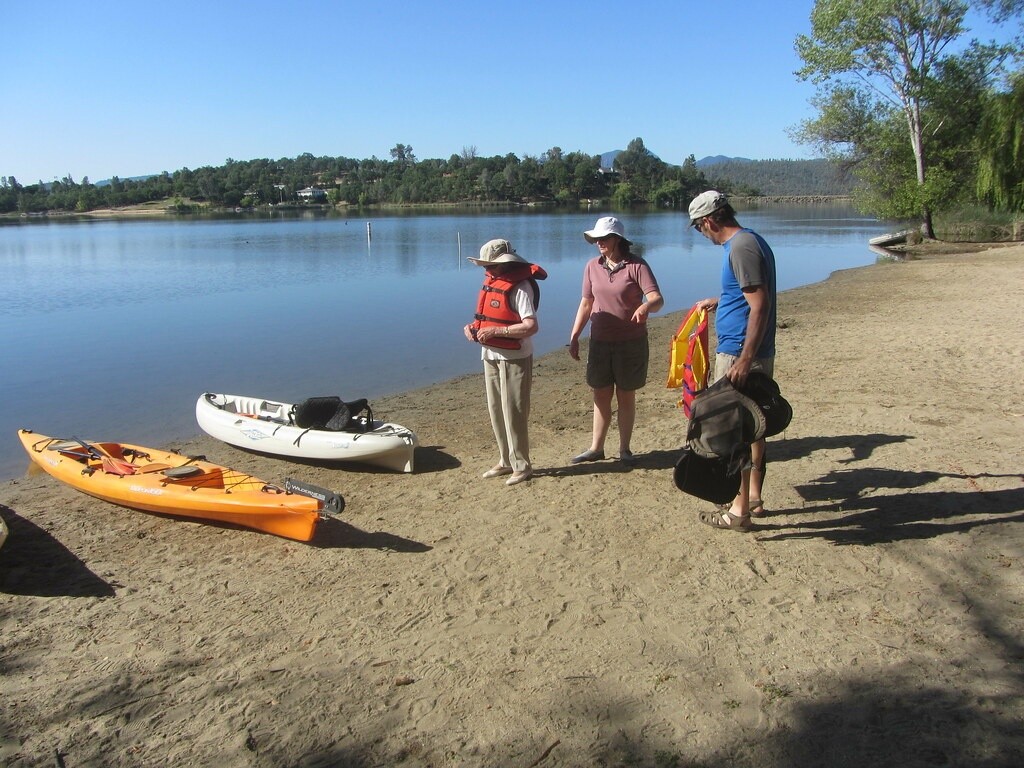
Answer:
[505,325,509,338]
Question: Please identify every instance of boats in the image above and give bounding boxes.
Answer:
[195,391,418,473]
[18,427,345,543]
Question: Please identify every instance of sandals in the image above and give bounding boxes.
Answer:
[715,497,764,518]
[699,507,753,533]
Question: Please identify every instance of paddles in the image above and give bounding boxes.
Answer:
[57,435,142,476]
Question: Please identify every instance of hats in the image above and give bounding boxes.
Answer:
[583,217,633,246]
[466,239,528,266]
[687,189,728,229]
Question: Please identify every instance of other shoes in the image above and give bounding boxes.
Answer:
[505,467,533,485]
[620,453,635,471]
[482,463,513,478]
[571,449,605,464]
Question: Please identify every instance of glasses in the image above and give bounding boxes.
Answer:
[593,235,616,243]
[483,264,498,270]
[694,215,708,232]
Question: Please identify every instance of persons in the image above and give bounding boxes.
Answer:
[569,217,664,465]
[683,190,778,531]
[464,239,539,486]
